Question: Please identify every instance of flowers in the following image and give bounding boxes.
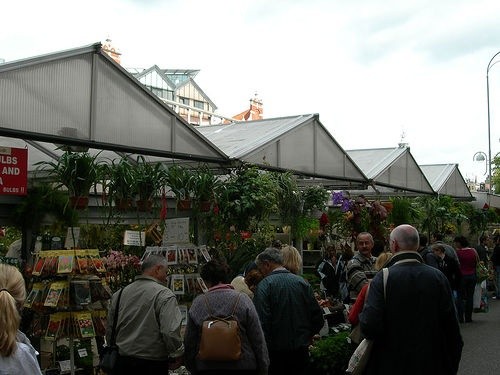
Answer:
[100,248,141,292]
[212,206,262,283]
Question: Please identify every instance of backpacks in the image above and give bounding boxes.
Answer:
[424,253,443,271]
[199,292,242,360]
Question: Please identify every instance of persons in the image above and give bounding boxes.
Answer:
[232,246,324,375]
[106,255,183,375]
[183,262,271,375]
[318,224,464,375]
[454,235,479,323]
[477,235,500,299]
[0,263,42,375]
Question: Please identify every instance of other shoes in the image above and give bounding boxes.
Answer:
[492,296,497,299]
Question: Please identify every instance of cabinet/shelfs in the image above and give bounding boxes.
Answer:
[302,250,321,268]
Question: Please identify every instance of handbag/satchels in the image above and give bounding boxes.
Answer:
[475,255,489,283]
[345,337,373,375]
[473,282,482,308]
[338,282,349,299]
[99,344,120,375]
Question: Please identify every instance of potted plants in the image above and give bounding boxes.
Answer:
[32,151,231,232]
